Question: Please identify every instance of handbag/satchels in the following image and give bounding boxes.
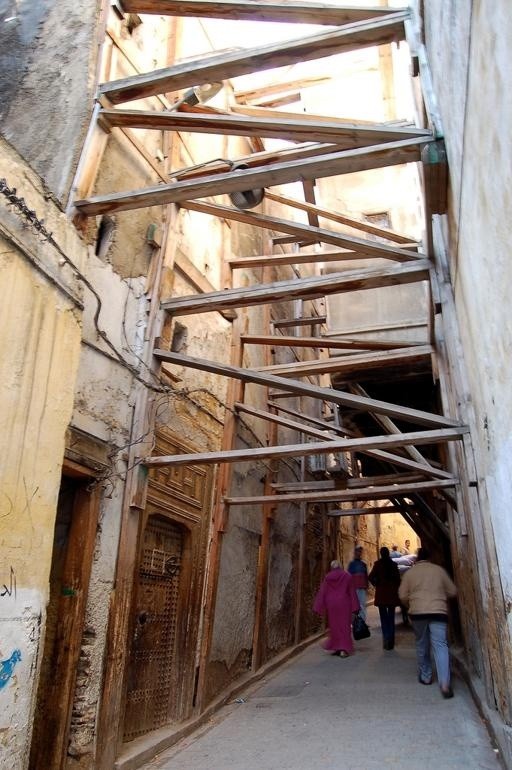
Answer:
[352,615,372,641]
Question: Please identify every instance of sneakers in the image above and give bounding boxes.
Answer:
[441,684,453,699]
[418,674,433,685]
[339,651,348,658]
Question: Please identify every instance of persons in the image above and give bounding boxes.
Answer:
[389,544,401,559]
[368,546,401,650]
[311,560,360,658]
[399,540,410,555]
[398,548,459,697]
[346,545,368,630]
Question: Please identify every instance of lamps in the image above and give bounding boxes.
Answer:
[166,154,269,211]
[161,87,201,115]
